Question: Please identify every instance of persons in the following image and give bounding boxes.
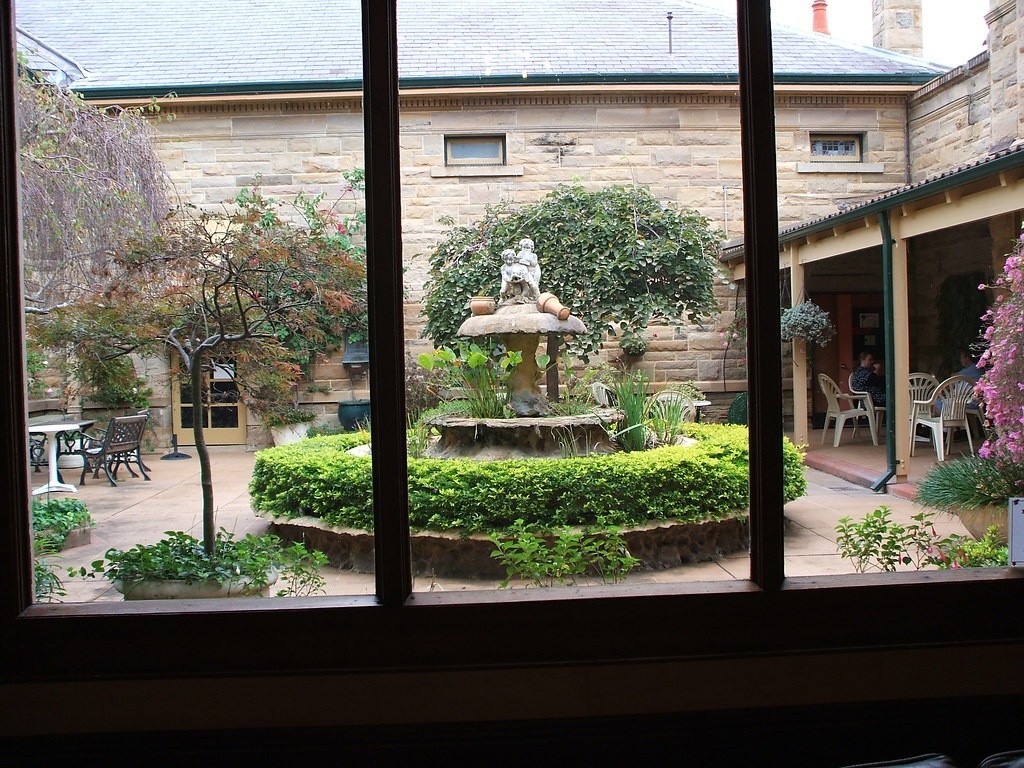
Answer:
[497,238,542,306]
[918,349,984,438]
[851,349,886,408]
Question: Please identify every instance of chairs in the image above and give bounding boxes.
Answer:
[592,382,619,409]
[849,373,887,439]
[818,373,879,448]
[647,390,697,423]
[908,372,989,463]
[726,392,749,425]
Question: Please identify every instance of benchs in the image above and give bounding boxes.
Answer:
[30,408,153,487]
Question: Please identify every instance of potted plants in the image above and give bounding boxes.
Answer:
[62,531,330,598]
[261,404,317,448]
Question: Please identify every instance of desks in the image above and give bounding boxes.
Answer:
[28,419,98,496]
[644,398,712,423]
[910,384,934,443]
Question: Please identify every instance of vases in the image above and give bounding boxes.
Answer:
[338,399,372,431]
[470,296,495,315]
